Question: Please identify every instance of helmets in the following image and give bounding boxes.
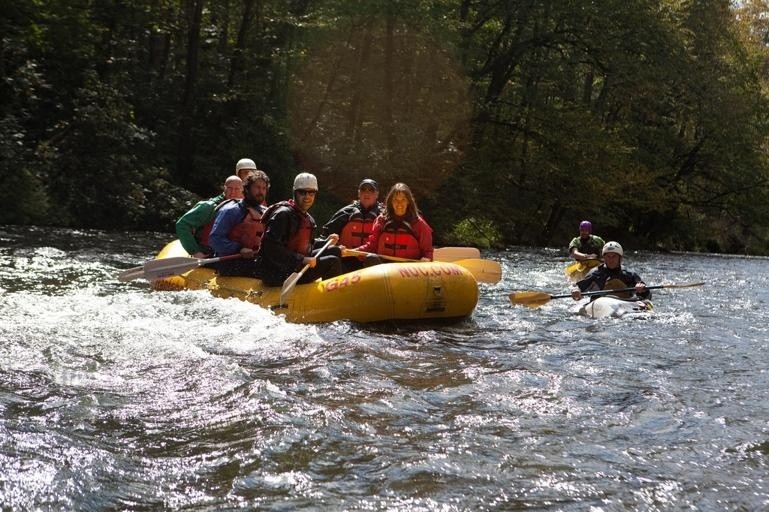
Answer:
[579,221,592,234]
[236,159,256,175]
[602,241,623,265]
[293,173,318,200]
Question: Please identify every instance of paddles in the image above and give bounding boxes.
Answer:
[509,280,708,308]
[144,252,257,281]
[279,237,335,305]
[432,246,480,263]
[342,249,502,284]
[118,266,146,284]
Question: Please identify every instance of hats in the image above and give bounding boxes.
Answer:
[357,179,379,189]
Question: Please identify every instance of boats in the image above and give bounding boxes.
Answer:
[565,258,603,279]
[570,293,655,321]
[579,294,654,320]
[568,261,603,283]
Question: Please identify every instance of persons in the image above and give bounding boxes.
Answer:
[341,182,433,268]
[255,172,341,286]
[322,178,384,271]
[570,240,652,302]
[569,220,605,261]
[175,175,243,269]
[207,170,271,277]
[236,158,266,206]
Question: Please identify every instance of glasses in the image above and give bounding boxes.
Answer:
[359,187,376,192]
[295,189,317,196]
